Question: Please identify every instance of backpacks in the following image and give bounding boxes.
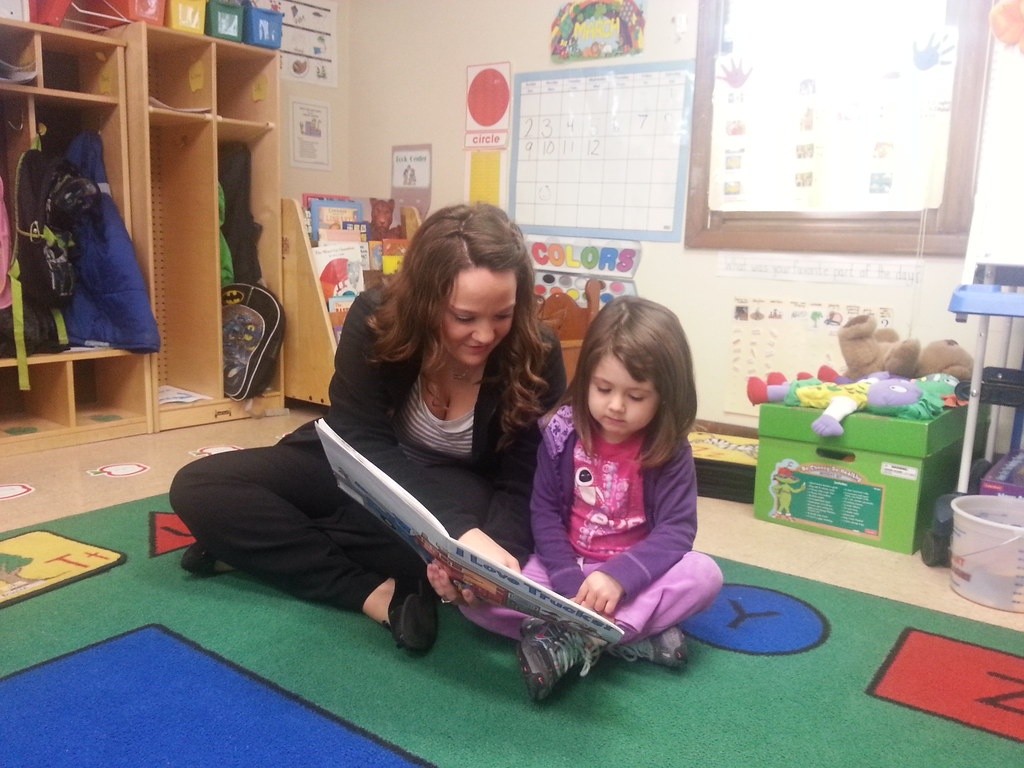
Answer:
[14,148,81,310]
[221,281,285,402]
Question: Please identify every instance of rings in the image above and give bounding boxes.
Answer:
[441,597,451,604]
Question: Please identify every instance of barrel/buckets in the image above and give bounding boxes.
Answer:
[950,497,1024,613]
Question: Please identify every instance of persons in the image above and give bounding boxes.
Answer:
[170,204,567,655]
[457,295,724,704]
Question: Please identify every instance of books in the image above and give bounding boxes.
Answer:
[301,193,410,346]
[315,418,626,649]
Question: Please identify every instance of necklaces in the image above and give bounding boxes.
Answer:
[453,365,470,379]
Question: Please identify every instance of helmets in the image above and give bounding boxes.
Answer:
[47,168,101,232]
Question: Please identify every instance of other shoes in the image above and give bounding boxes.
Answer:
[383,575,438,655]
[180,541,235,576]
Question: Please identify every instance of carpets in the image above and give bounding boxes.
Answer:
[0,493,1024,768]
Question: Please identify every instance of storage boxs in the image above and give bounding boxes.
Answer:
[753,400,990,556]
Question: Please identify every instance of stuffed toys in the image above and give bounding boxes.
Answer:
[747,314,973,436]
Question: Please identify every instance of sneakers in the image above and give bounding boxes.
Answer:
[606,625,685,667]
[519,616,606,701]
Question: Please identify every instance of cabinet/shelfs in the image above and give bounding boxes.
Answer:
[101,21,284,430]
[0,19,154,456]
